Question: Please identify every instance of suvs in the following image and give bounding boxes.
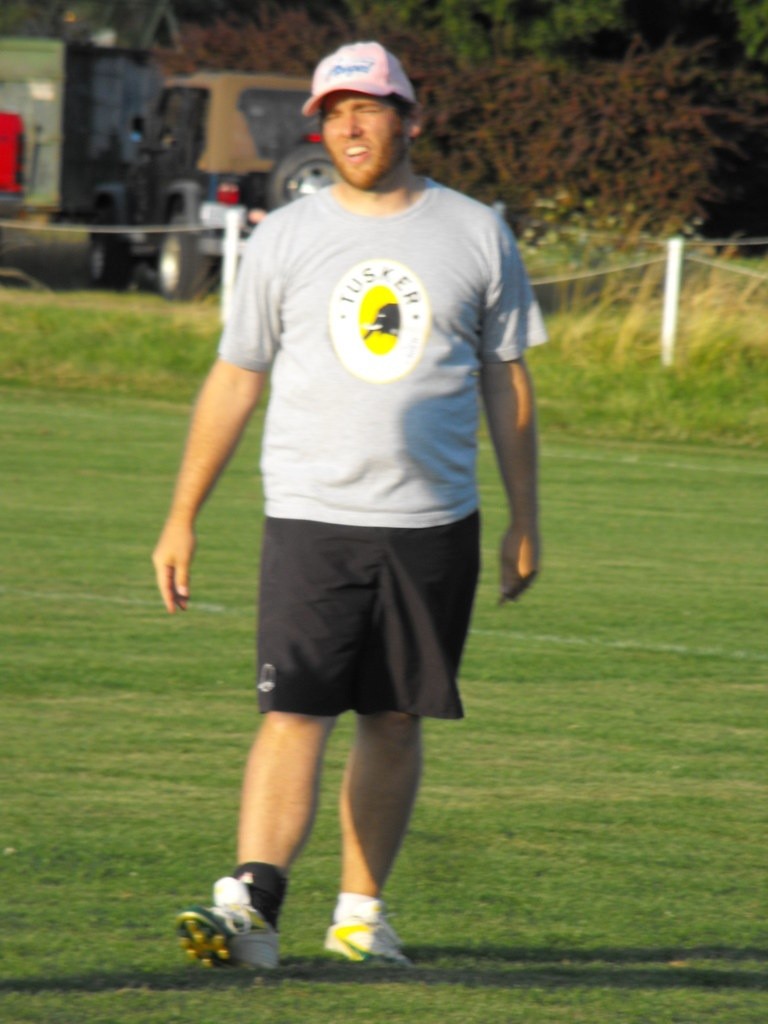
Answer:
[0,0,181,225]
[82,72,423,302]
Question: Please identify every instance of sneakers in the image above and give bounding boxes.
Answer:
[324,916,413,973]
[174,876,280,973]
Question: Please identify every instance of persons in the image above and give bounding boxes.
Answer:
[149,40,546,973]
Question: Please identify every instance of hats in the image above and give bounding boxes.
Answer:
[302,41,417,118]
